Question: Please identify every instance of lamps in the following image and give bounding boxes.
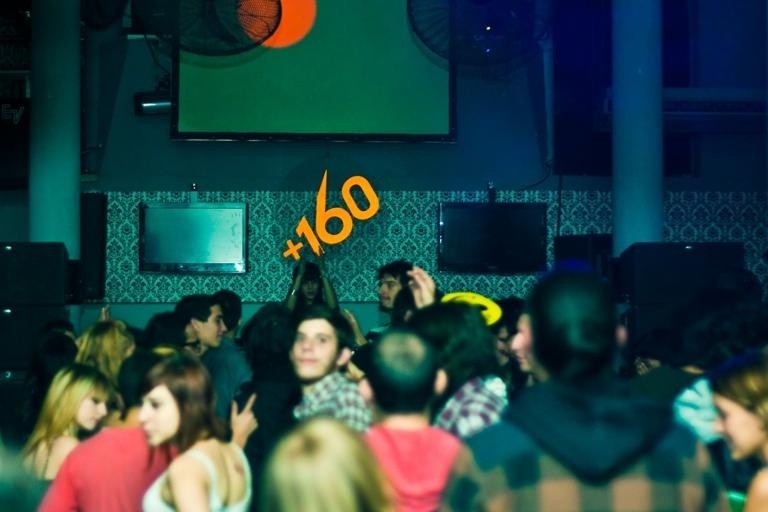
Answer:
[134,24,175,116]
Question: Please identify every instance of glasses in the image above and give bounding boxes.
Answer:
[182,339,202,352]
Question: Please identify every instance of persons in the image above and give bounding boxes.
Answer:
[0,245,767,512]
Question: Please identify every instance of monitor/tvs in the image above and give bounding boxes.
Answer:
[437,201,547,275]
[137,200,248,278]
